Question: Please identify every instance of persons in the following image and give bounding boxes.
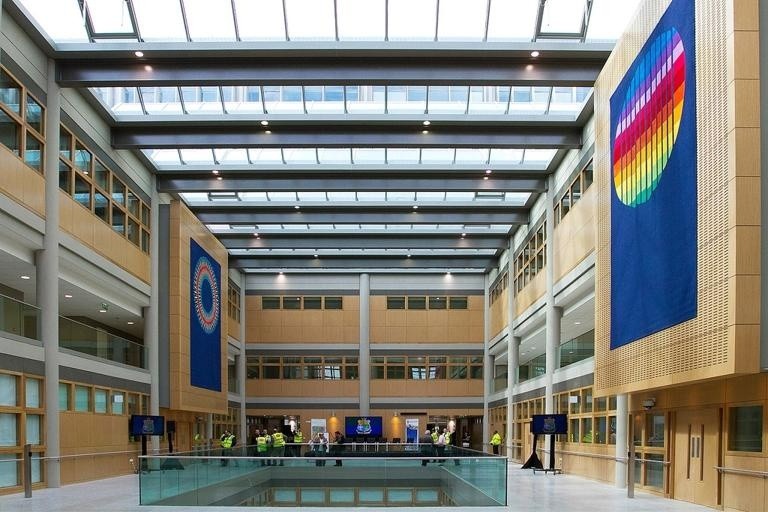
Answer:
[219,429,237,467]
[462,432,472,449]
[294,428,304,457]
[489,430,501,455]
[313,432,328,466]
[251,428,288,466]
[333,432,345,467]
[419,427,451,466]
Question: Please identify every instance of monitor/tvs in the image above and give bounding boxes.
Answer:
[130,415,164,437]
[532,415,568,434]
[343,416,383,437]
